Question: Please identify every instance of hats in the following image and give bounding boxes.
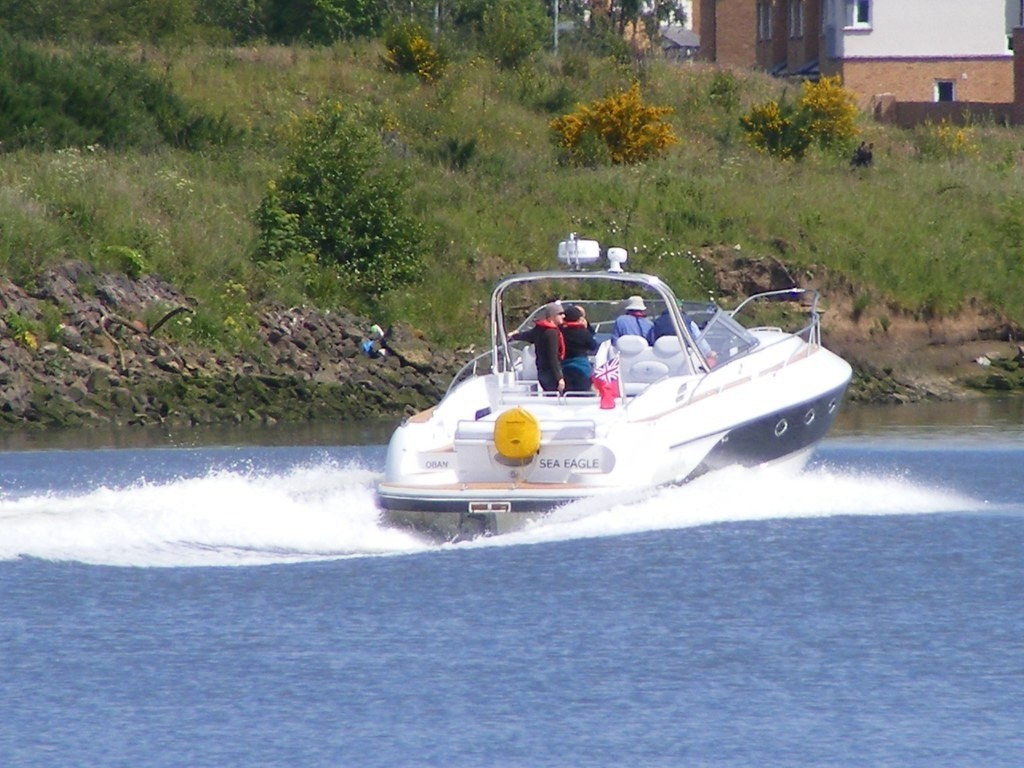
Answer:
[624,296,647,310]
[675,298,683,307]
[545,303,564,318]
[564,306,583,321]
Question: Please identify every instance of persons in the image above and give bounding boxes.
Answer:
[559,307,597,397]
[508,302,566,396]
[647,299,717,359]
[611,295,654,346]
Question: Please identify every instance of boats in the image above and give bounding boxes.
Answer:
[378,232,852,541]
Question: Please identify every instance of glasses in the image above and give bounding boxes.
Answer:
[558,311,565,315]
